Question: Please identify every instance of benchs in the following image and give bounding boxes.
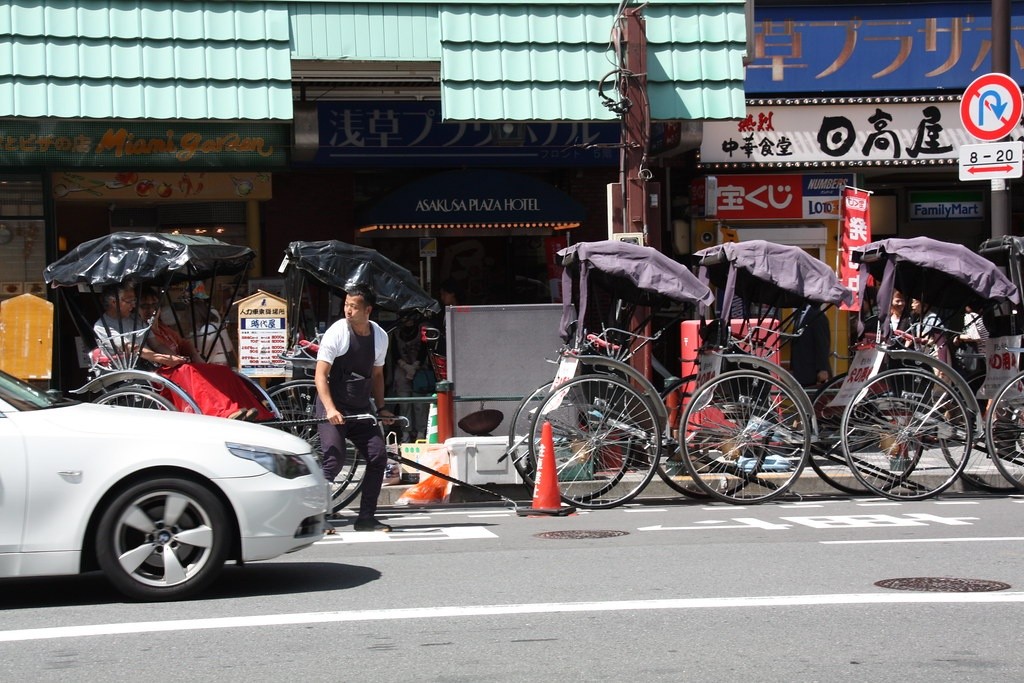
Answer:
[566,320,660,430]
[701,322,774,406]
[952,354,989,421]
[62,288,140,371]
[861,317,934,409]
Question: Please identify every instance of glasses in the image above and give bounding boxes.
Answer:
[121,299,137,305]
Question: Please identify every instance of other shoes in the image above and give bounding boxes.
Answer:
[325,521,336,535]
[354,519,392,532]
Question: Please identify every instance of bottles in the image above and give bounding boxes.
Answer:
[317,321,326,340]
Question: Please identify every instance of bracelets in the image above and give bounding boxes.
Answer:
[376,406,388,415]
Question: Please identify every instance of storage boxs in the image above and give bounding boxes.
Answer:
[445,435,528,487]
[531,438,594,483]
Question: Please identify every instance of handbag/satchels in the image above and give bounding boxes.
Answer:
[952,341,978,371]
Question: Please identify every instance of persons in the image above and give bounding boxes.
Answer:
[314,282,394,536]
[94,277,259,421]
[789,300,835,437]
[881,284,993,444]
[390,277,460,443]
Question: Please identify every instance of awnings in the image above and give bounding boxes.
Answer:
[0,0,748,119]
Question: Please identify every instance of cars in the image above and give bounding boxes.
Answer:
[0,369,335,603]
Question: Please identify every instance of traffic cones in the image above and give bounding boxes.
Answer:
[514,422,580,519]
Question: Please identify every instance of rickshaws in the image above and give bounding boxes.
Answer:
[39,230,412,527]
[262,239,522,512]
[508,235,1024,510]
